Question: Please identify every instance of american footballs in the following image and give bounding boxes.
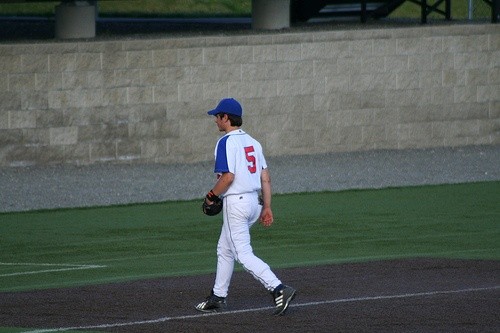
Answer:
[202,197,223,216]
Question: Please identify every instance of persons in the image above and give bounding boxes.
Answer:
[195,98,297,317]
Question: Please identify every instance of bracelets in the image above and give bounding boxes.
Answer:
[206,190,219,201]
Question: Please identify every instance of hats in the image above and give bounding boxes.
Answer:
[207,98,242,115]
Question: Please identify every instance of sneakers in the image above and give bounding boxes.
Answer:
[273,285,297,316]
[195,297,227,312]
